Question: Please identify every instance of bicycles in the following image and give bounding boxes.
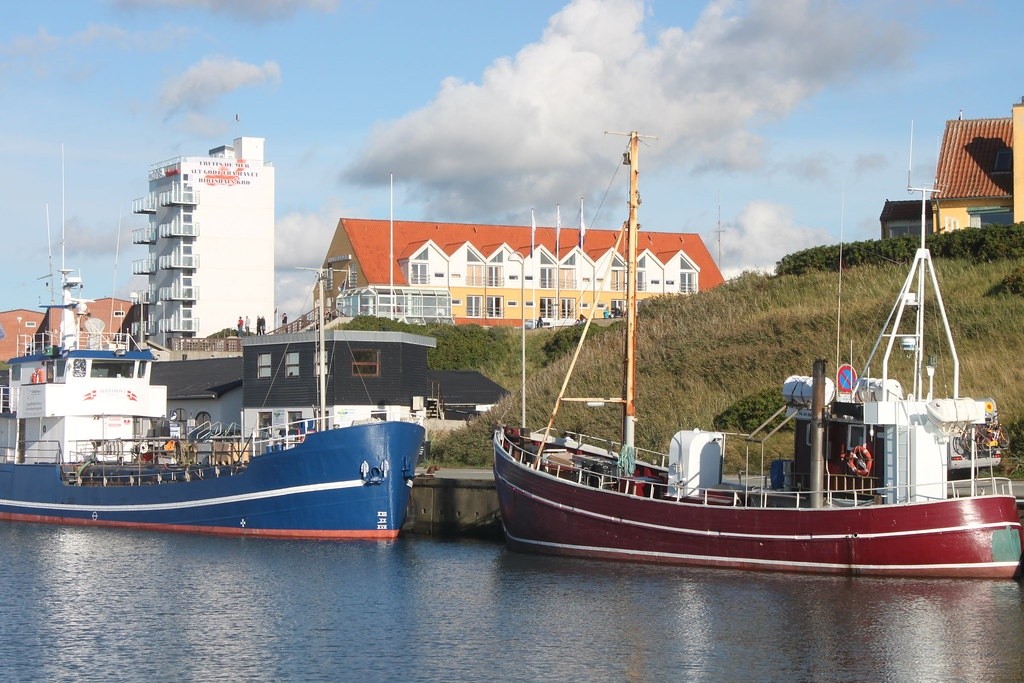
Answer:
[952,410,1010,454]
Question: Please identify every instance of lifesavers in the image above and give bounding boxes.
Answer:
[848,445,872,476]
[32,369,44,382]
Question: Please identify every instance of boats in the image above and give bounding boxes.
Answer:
[0,144,428,544]
[493,128,1021,579]
